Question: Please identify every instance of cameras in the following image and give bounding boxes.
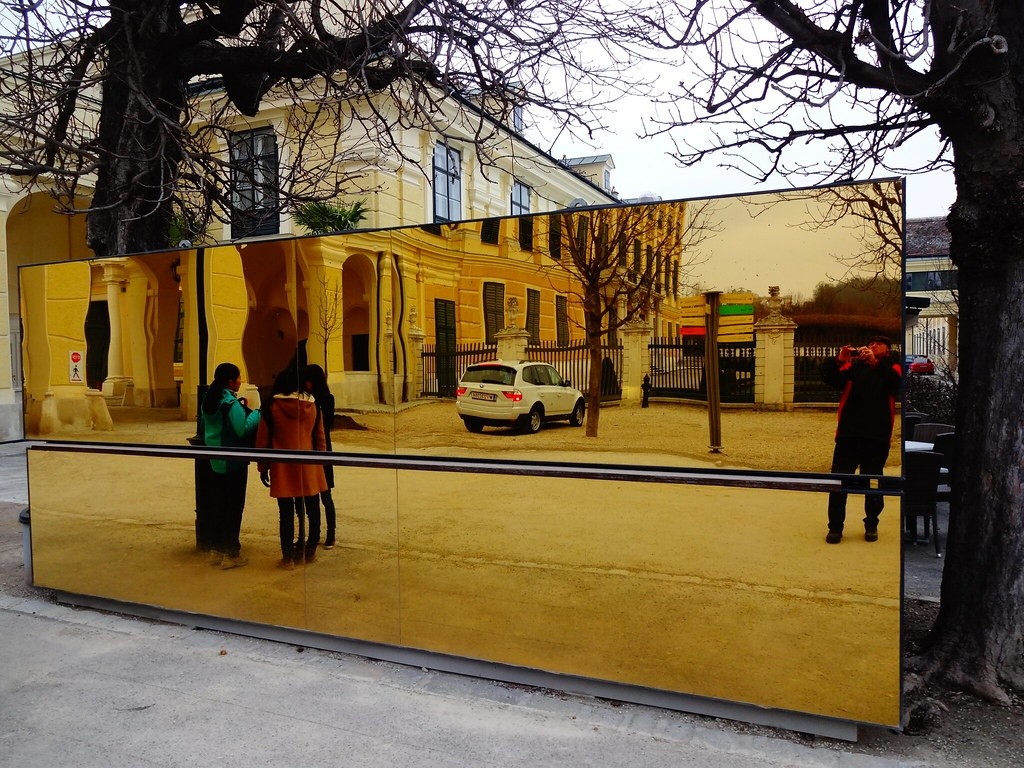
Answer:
[850,350,861,356]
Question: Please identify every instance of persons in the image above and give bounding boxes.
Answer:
[255,369,327,569]
[824,339,903,544]
[199,363,261,568]
[307,365,337,549]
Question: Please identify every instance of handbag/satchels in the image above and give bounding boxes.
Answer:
[221,397,259,464]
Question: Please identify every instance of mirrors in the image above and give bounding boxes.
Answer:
[24,446,906,730]
[17,178,905,479]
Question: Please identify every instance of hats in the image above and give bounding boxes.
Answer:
[866,335,892,351]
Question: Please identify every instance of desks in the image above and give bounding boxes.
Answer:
[905,441,935,453]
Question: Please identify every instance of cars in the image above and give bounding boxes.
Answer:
[906,355,925,372]
[909,357,934,375]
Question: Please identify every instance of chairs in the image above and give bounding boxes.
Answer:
[923,432,954,542]
[904,451,943,558]
[903,412,954,443]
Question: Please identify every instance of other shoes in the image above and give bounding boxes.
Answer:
[305,553,318,563]
[322,538,336,548]
[277,552,305,569]
[864,527,878,541]
[826,529,842,543]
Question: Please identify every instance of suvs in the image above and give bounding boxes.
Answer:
[456,359,586,433]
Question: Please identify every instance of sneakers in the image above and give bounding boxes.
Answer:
[209,550,248,570]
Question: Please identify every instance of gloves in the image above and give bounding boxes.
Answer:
[261,471,270,488]
